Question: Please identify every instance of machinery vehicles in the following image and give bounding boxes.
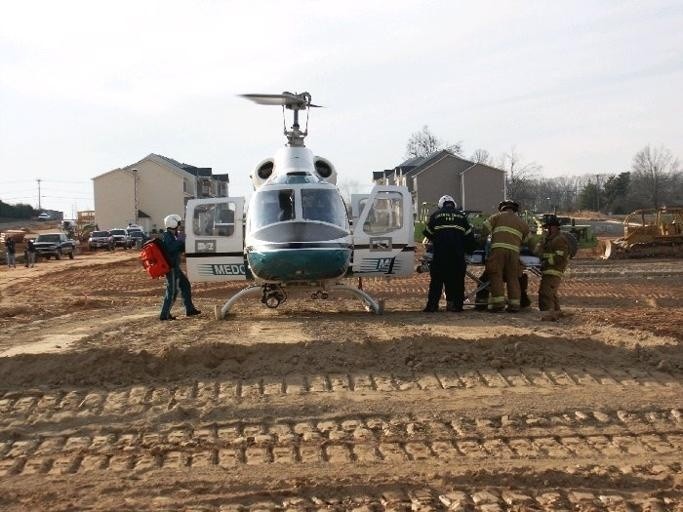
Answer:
[602,204,683,260]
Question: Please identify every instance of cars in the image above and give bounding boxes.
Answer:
[126,223,144,247]
[558,216,591,241]
[38,212,50,221]
[88,231,113,248]
[108,228,128,246]
[33,233,76,260]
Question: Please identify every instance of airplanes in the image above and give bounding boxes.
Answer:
[179,90,417,322]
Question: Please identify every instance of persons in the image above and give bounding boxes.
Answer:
[126,223,132,236]
[5,237,16,268]
[538,214,569,312]
[152,224,157,234]
[24,239,36,268]
[482,199,529,313]
[159,214,201,320]
[159,224,164,233]
[421,195,473,313]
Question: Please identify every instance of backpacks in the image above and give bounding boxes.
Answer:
[139,237,171,280]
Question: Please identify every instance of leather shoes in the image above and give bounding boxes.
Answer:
[451,306,463,312]
[187,311,202,316]
[159,317,177,321]
[424,306,436,312]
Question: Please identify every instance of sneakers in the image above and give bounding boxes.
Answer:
[506,307,520,314]
[556,311,564,318]
[489,307,506,313]
[541,316,554,322]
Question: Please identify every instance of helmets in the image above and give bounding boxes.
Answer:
[438,195,458,209]
[542,215,560,227]
[164,214,182,229]
[497,198,519,211]
